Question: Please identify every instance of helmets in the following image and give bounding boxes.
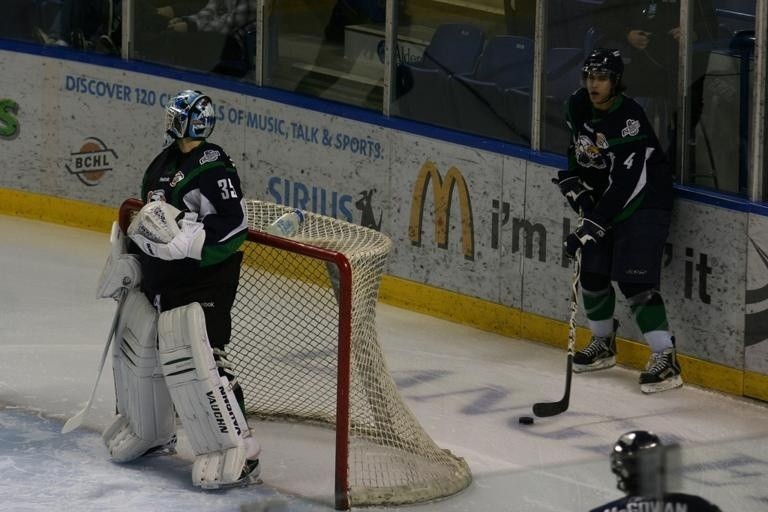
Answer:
[581,50,620,100]
[610,431,664,496]
[165,89,215,140]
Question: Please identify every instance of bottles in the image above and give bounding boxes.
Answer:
[267,208,305,237]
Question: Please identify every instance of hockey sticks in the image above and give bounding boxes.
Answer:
[61,287,129,436]
[533,205,585,418]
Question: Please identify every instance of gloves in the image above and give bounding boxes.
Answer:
[562,218,606,257]
[551,170,590,213]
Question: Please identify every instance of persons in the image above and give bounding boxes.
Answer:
[97,88,262,492]
[558,48,685,393]
[592,430,726,512]
[592,0,719,190]
[35,0,257,78]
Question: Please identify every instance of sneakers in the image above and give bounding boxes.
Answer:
[574,319,619,364]
[237,457,260,478]
[640,337,680,383]
[144,435,177,455]
